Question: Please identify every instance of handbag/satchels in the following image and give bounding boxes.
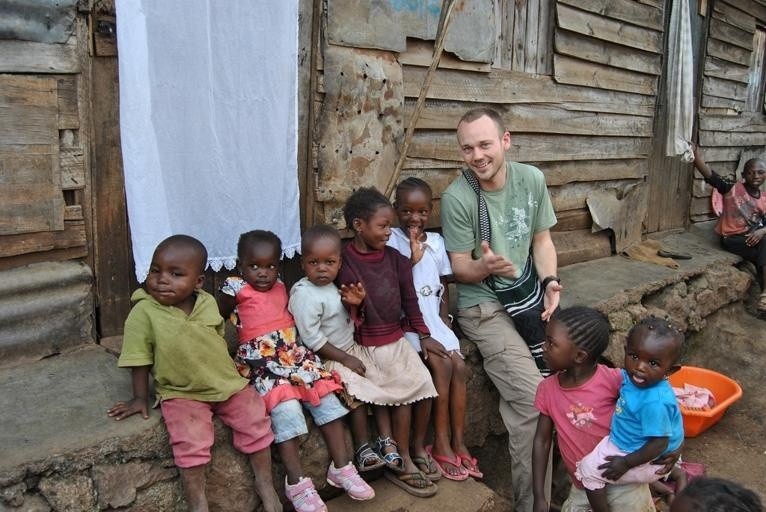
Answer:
[495,255,561,378]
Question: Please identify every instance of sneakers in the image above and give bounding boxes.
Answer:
[285,475,328,512]
[327,460,375,500]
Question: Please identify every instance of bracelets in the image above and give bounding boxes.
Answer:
[418,334,430,340]
[541,275,560,286]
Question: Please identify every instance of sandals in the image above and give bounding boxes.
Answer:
[376,437,405,472]
[756,293,766,310]
[354,443,386,471]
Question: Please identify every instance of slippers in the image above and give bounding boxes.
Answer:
[386,443,483,497]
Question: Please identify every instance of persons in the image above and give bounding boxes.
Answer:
[531,306,685,512]
[668,475,766,512]
[336,185,451,496]
[574,318,684,512]
[688,144,766,313]
[441,110,563,512]
[108,235,283,512]
[218,230,375,512]
[388,177,484,482]
[288,225,407,473]
